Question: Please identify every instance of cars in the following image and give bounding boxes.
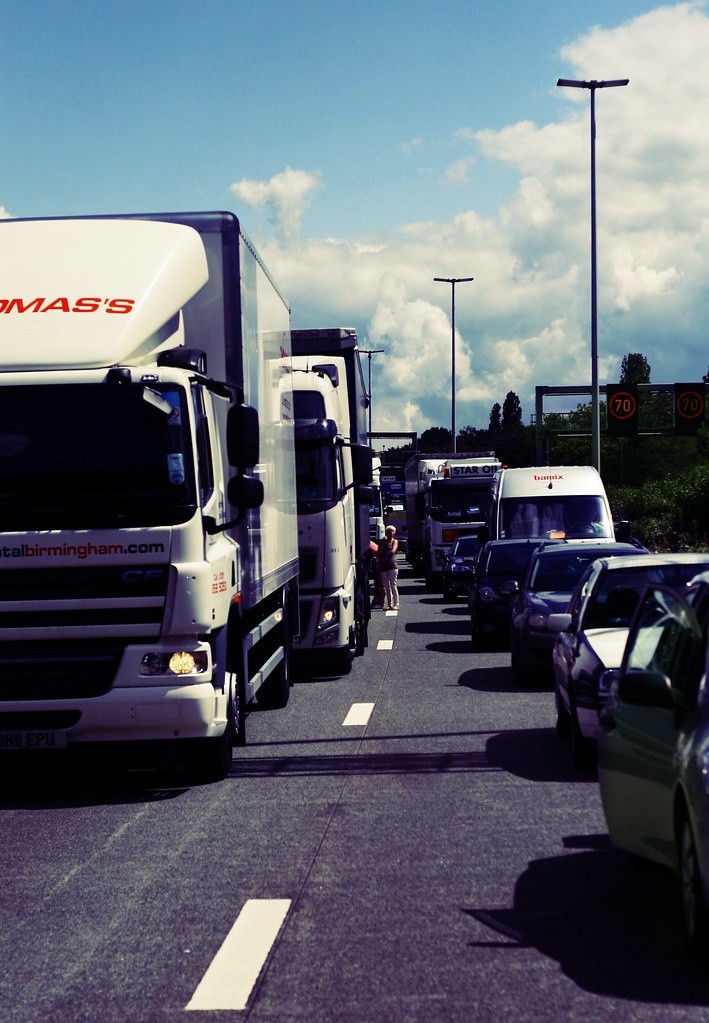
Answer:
[542,553,709,758]
[443,537,483,603]
[465,540,572,645]
[506,542,662,688]
[590,570,709,901]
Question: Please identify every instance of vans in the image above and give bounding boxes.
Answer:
[486,464,615,568]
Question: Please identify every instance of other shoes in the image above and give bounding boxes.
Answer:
[384,606,392,610]
[392,606,399,610]
[371,602,383,609]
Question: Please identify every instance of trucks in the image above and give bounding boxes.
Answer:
[405,458,505,589]
[291,329,393,676]
[0,212,298,778]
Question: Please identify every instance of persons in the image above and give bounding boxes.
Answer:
[375,525,402,610]
[583,506,604,536]
[369,539,385,610]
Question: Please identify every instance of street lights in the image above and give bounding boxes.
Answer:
[561,78,632,474]
[436,277,472,449]
